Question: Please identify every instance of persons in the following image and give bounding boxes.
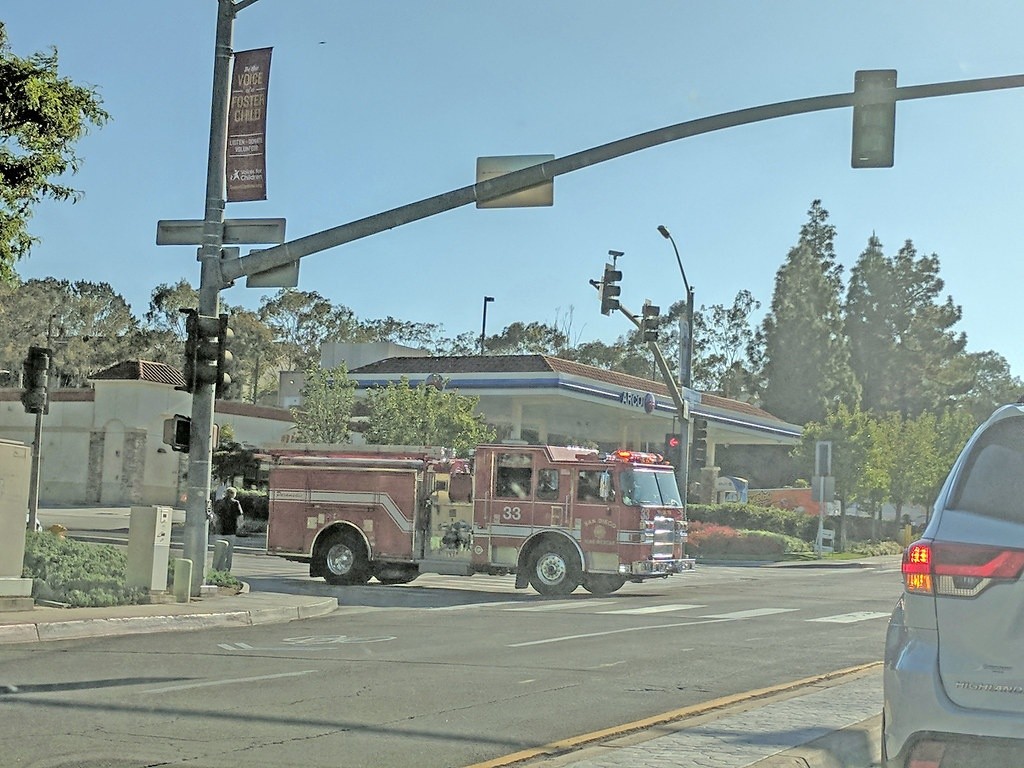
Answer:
[578,471,603,501]
[212,486,243,573]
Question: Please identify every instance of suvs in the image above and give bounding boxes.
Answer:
[883,401,1024,768]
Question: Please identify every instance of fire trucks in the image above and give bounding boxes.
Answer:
[264,443,696,596]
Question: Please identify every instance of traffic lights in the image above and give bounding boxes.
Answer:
[601,263,622,316]
[666,433,682,470]
[643,302,660,345]
[162,416,190,448]
[217,313,234,390]
[693,417,706,471]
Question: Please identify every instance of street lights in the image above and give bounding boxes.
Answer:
[481,296,495,352]
[658,225,695,523]
